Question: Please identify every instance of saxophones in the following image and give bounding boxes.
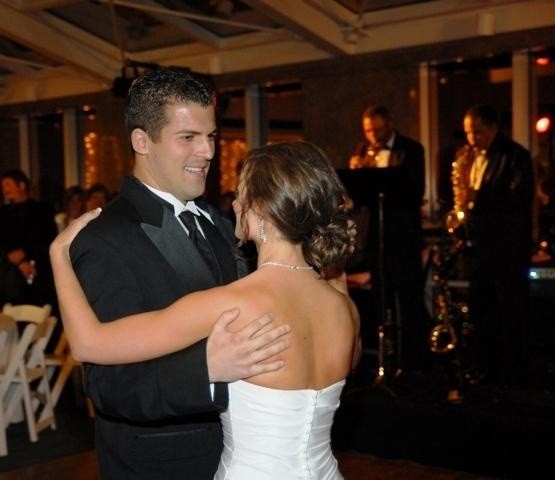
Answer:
[429,272,458,354]
[442,143,478,238]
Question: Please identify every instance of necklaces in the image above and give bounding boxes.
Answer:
[256,261,319,273]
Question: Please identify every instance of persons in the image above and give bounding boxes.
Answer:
[349,104,425,203]
[69,64,292,480]
[49,139,360,480]
[452,100,534,280]
[55,181,111,233]
[0,165,58,308]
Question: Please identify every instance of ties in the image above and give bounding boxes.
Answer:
[179,211,222,286]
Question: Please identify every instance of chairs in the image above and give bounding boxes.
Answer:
[0,314,14,457]
[0,302,57,441]
[31,329,96,433]
